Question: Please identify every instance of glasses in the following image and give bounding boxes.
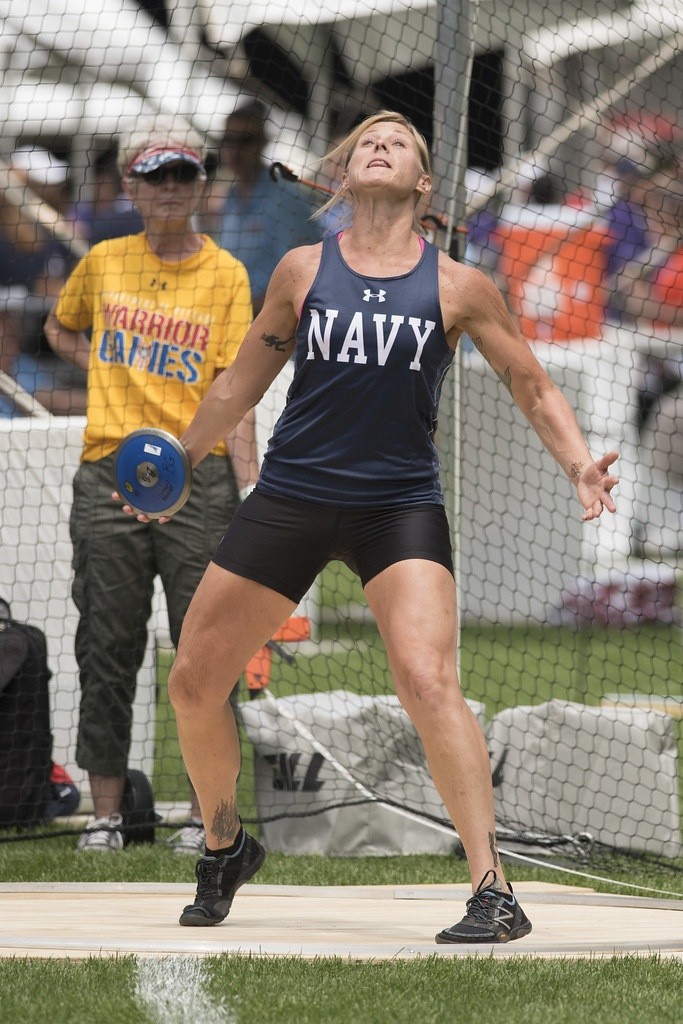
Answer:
[129,161,200,186]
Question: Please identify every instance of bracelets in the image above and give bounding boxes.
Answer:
[239,484,256,501]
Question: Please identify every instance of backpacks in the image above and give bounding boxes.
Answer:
[47,762,80,815]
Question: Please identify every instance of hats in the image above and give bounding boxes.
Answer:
[126,139,207,185]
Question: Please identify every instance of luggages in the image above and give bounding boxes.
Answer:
[0,598,52,831]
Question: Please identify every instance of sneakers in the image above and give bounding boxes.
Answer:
[75,814,124,852]
[434,868,533,944]
[167,816,206,857]
[180,828,267,926]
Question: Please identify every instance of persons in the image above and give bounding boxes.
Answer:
[0,147,144,418]
[45,120,259,851]
[112,112,619,943]
[221,102,318,321]
[466,136,683,336]
